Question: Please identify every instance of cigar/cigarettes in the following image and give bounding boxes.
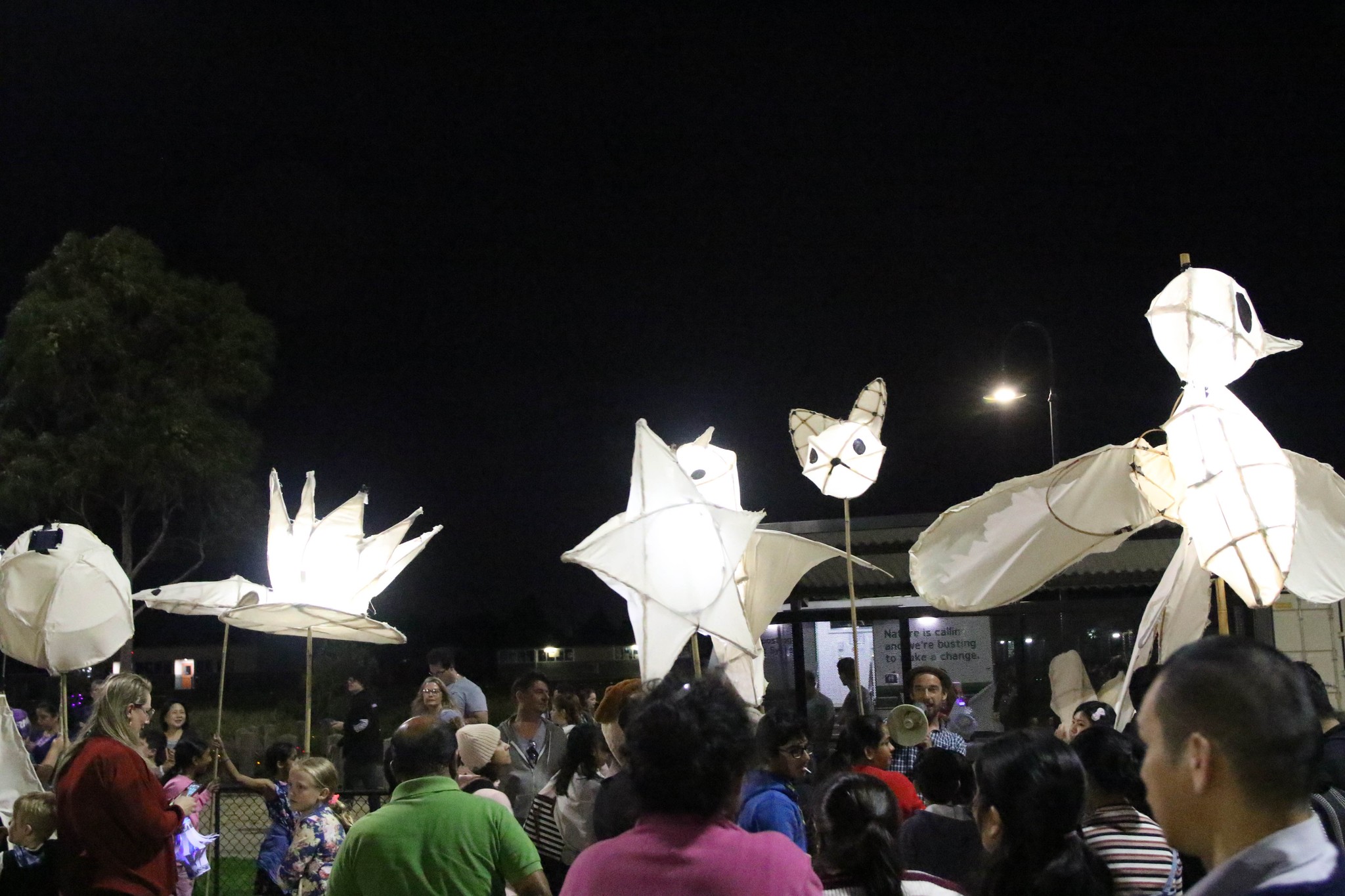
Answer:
[803,767,812,774]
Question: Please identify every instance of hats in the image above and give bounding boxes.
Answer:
[904,664,953,700]
[455,724,500,771]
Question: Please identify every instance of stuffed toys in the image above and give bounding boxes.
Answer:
[594,678,646,773]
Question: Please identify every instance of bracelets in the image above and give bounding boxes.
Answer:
[222,758,231,763]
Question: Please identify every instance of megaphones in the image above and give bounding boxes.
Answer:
[887,702,928,746]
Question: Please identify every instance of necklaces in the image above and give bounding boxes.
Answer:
[454,672,459,683]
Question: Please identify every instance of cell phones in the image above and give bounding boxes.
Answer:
[168,781,205,806]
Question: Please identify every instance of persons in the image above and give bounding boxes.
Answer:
[557,671,824,896]
[972,727,1115,896]
[408,647,620,896]
[210,731,303,896]
[326,715,551,895]
[53,672,198,896]
[328,671,383,813]
[0,679,220,896]
[276,757,354,896]
[735,657,1344,896]
[1136,637,1345,896]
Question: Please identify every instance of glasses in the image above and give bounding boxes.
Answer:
[529,740,536,761]
[133,704,155,718]
[778,741,816,759]
[422,688,441,695]
[429,668,449,677]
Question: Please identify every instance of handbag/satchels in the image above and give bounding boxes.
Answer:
[523,793,564,863]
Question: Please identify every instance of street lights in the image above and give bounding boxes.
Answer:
[984,379,1060,464]
